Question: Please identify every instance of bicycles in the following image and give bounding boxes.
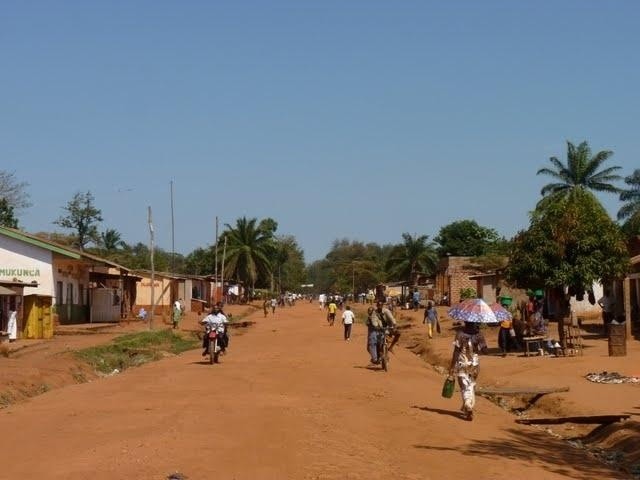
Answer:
[374,326,393,370]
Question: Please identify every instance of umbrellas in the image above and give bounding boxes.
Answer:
[488,303,513,321]
[446,297,499,323]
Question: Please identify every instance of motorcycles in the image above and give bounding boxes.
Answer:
[202,322,227,363]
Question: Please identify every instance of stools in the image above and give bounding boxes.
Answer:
[524,334,545,357]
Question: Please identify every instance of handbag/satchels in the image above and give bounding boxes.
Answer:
[442,377,455,398]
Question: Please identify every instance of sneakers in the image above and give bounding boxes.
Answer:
[461,404,473,421]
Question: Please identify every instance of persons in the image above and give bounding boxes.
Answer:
[422,300,439,339]
[598,288,620,333]
[263,290,314,317]
[172,298,186,330]
[557,294,579,356]
[497,298,548,357]
[198,301,233,357]
[448,321,488,421]
[317,288,420,365]
[440,294,449,306]
